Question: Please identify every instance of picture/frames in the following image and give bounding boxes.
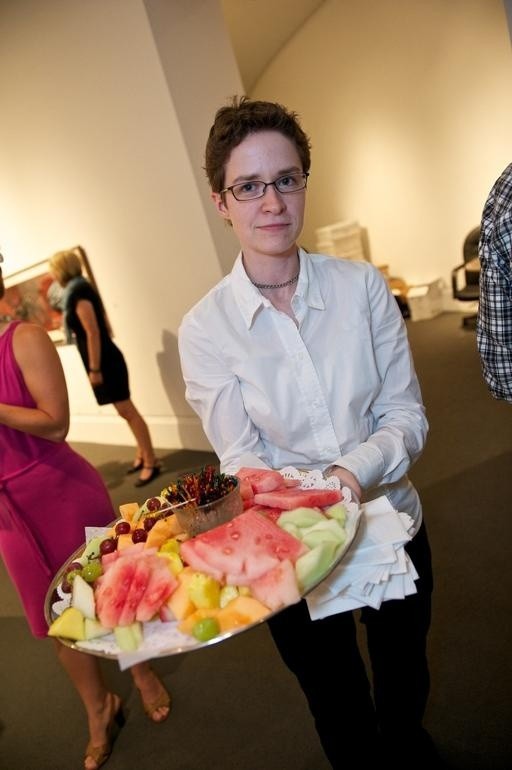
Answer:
[0,245,113,348]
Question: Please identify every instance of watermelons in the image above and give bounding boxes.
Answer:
[94,468,341,631]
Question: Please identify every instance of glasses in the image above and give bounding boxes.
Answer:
[220,172,309,201]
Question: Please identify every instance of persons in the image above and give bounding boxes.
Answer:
[477,163,512,402]
[178,101,432,770]
[0,266,172,770]
[45,251,160,488]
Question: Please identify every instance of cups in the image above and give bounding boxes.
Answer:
[166,473,245,537]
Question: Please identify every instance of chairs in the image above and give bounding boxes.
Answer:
[452,226,481,325]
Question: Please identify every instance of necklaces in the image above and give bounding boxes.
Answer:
[251,272,299,288]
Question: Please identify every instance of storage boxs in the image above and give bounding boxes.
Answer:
[315,221,447,322]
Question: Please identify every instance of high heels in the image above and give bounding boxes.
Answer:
[142,688,170,722]
[127,459,160,487]
[83,693,126,770]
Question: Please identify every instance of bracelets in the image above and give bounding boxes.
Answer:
[89,368,101,371]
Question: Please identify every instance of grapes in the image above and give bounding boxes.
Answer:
[192,617,216,640]
[62,498,170,592]
